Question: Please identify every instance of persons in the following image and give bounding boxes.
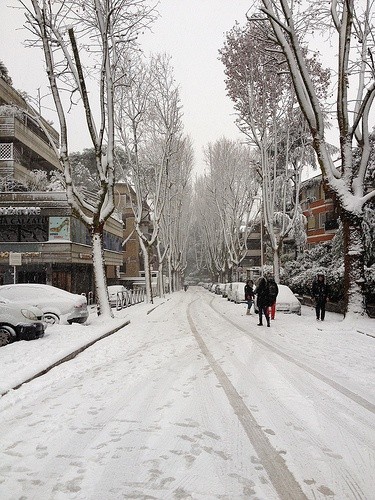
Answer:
[245,280,255,315]
[254,277,270,327]
[312,272,328,320]
[267,277,278,319]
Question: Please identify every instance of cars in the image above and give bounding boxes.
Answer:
[0,296,48,347]
[106,285,131,308]
[197,282,257,304]
[0,283,89,325]
[252,285,302,316]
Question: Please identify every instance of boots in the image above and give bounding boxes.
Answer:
[266,317,270,327]
[246,309,252,315]
[257,314,263,326]
